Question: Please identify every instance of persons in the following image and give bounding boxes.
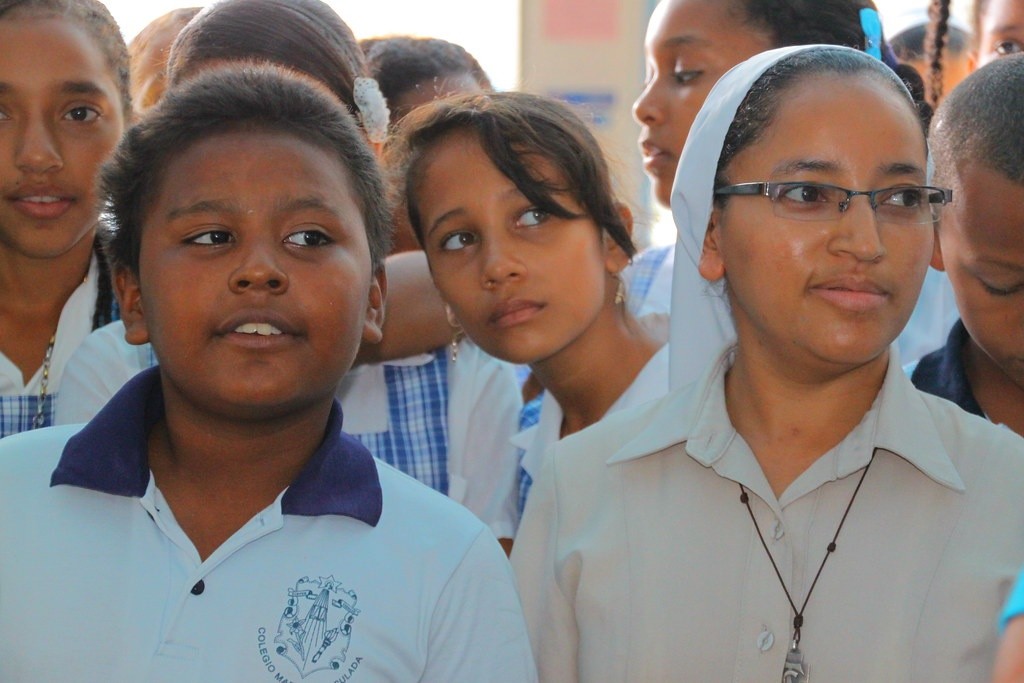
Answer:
[2,0,1022,681]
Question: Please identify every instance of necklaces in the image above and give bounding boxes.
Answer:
[739,448,880,683]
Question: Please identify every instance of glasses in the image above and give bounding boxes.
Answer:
[716,180,954,225]
